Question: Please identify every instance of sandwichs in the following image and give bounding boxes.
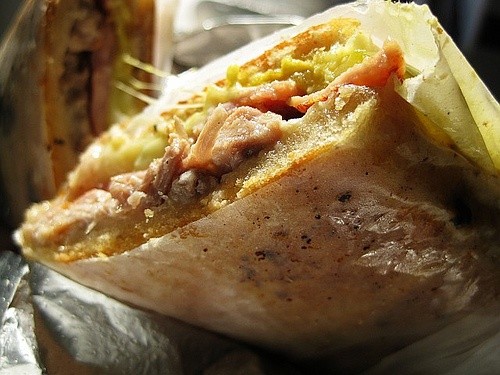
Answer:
[10,17,499,351]
